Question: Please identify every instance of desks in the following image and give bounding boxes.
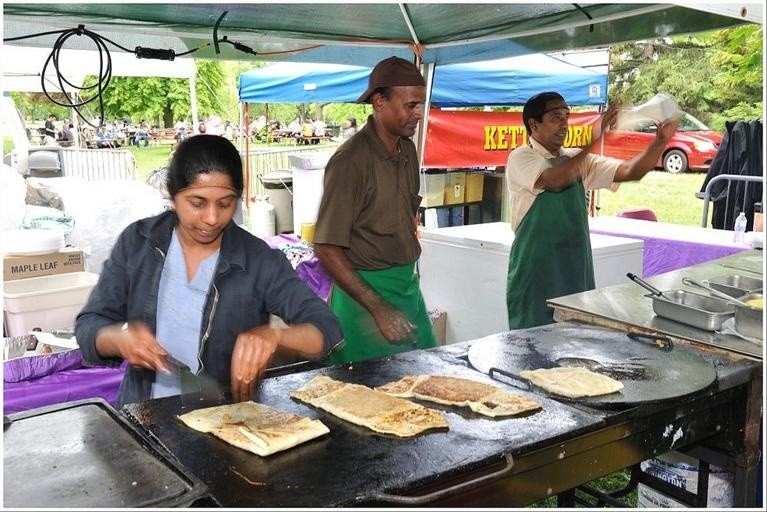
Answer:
[4,359,132,417]
[588,213,763,277]
[541,244,763,508]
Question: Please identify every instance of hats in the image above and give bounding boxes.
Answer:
[357,56,425,103]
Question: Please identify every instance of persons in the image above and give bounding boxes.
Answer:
[506,91,678,331]
[73,135,344,410]
[170,113,359,146]
[311,57,439,363]
[42,113,155,150]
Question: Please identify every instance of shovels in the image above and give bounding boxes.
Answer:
[158,353,224,405]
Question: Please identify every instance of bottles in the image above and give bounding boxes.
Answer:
[733,212,747,245]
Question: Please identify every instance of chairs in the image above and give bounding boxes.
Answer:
[617,208,659,222]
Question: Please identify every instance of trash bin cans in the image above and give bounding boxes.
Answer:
[256,171,294,234]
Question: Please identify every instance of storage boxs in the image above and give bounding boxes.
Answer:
[417,167,487,228]
[3,267,101,337]
[2,246,87,280]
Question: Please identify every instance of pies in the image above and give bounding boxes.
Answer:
[176,401,331,458]
[522,363,626,399]
[371,372,542,417]
[292,371,452,439]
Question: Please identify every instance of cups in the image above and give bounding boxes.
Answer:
[300,223,316,244]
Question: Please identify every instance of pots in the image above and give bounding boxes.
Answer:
[255,169,296,189]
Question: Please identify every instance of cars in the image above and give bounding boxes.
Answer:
[96,131,120,149]
[251,118,300,138]
[587,104,725,176]
[121,131,149,146]
